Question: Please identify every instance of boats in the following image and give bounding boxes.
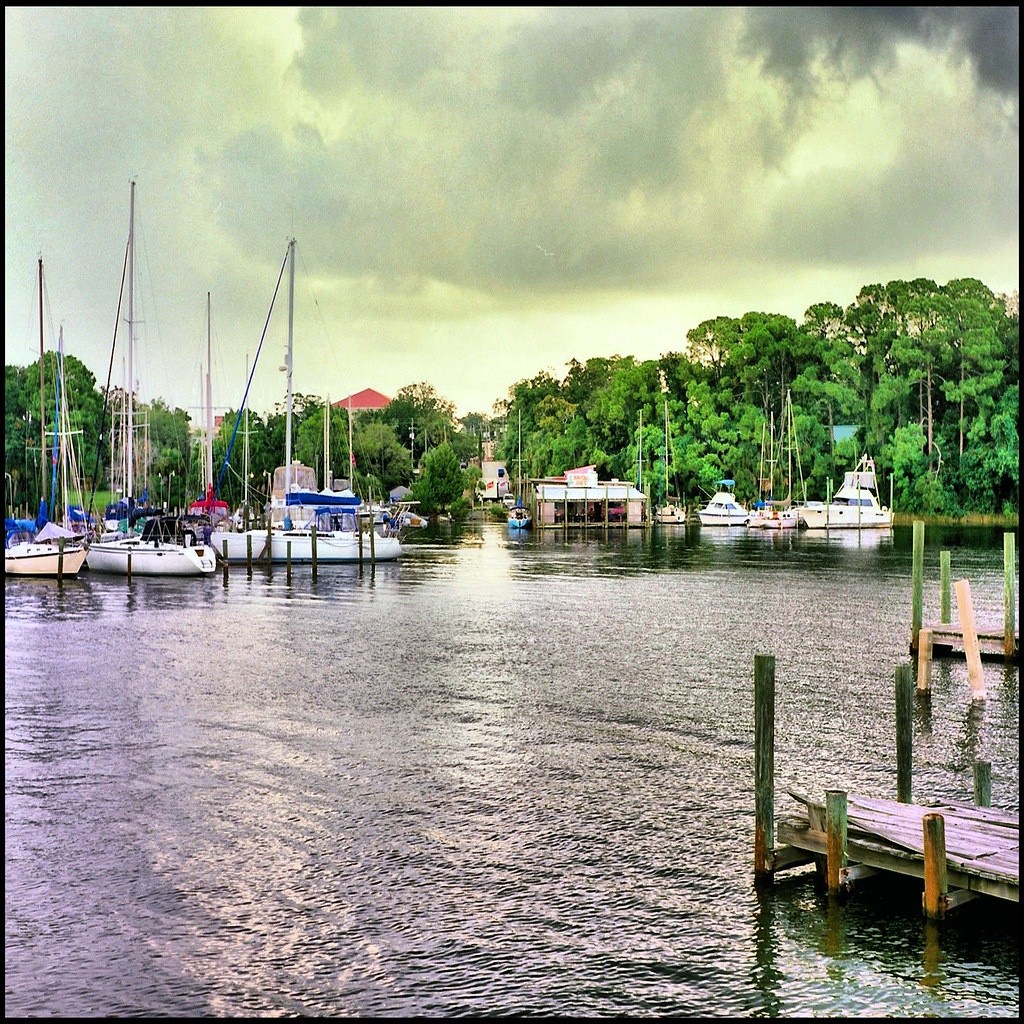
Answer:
[797,452,895,530]
[697,480,751,526]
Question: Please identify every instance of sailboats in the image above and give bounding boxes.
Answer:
[748,389,805,528]
[86,180,217,575]
[18,320,396,542]
[508,408,531,527]
[654,402,687,522]
[4,252,95,578]
[209,233,402,562]
